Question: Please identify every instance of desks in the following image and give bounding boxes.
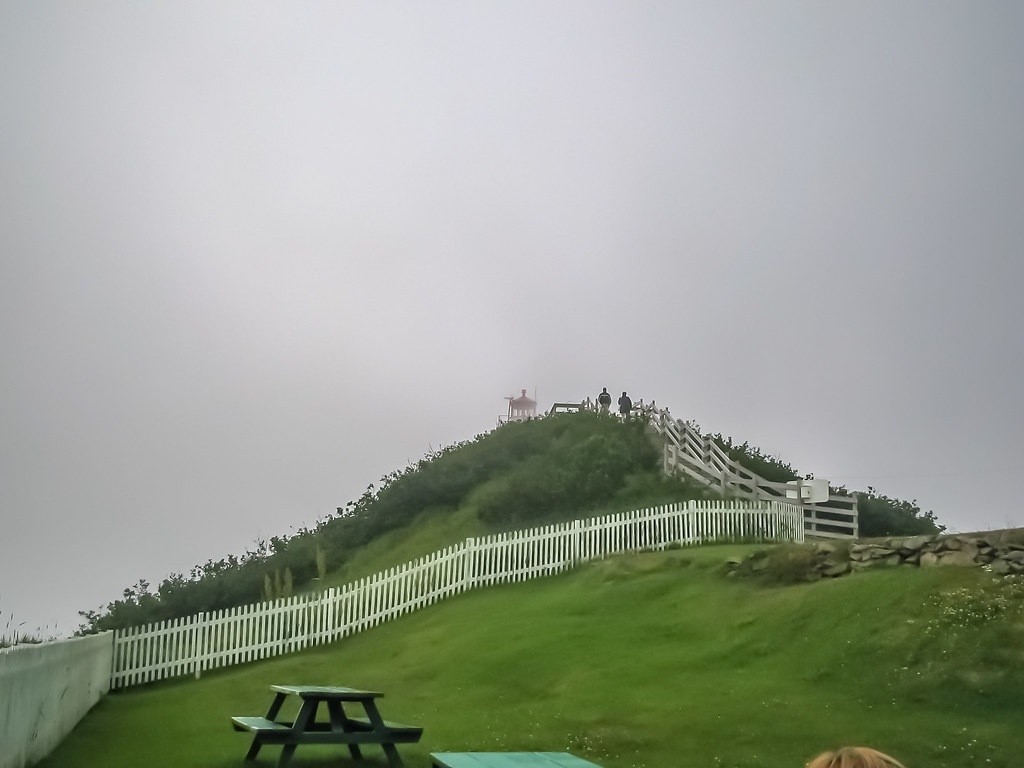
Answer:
[427,750,602,768]
[245,684,404,768]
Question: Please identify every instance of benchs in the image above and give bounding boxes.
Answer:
[231,716,292,737]
[347,718,423,743]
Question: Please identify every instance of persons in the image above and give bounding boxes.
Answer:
[803,746,902,767]
[617,391,633,420]
[597,387,612,412]
